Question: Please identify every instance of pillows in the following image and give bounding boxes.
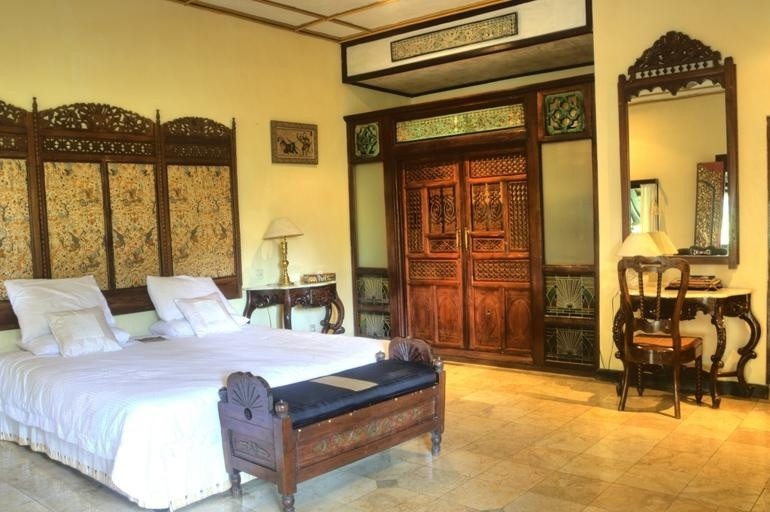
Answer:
[146,275,237,322]
[150,313,249,339]
[174,291,243,339]
[43,303,123,358]
[4,275,115,344]
[12,326,130,356]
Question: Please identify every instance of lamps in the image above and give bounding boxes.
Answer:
[649,231,679,255]
[263,216,304,287]
[618,232,662,290]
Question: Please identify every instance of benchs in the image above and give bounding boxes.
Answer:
[216,336,445,512]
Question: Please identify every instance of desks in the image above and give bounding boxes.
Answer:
[244,281,345,334]
[612,287,761,409]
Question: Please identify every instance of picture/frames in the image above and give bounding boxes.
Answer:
[271,120,318,165]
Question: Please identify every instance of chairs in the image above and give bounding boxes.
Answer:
[617,256,703,418]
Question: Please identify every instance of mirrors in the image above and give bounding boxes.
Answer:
[617,31,740,270]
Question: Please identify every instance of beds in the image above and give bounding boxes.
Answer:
[0,323,392,512]
